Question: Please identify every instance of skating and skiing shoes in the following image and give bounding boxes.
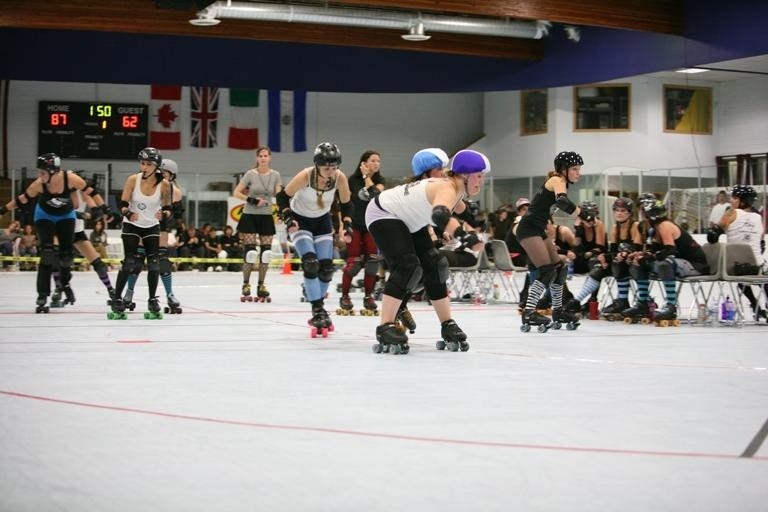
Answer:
[239,277,274,304]
[600,297,683,327]
[32,285,76,313]
[305,304,337,339]
[549,306,581,331]
[335,292,357,318]
[434,317,472,354]
[370,322,413,355]
[162,290,185,316]
[360,294,383,317]
[103,288,138,320]
[142,297,165,320]
[518,308,556,335]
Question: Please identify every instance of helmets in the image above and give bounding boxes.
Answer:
[37,150,62,179]
[311,141,345,168]
[137,145,179,179]
[554,146,756,221]
[411,146,452,178]
[449,146,492,175]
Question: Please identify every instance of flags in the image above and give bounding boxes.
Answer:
[149,84,182,149]
[266,89,308,153]
[189,86,220,147]
[227,86,259,149]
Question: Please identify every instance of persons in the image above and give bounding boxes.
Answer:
[110,145,174,315]
[363,149,491,346]
[372,147,476,302]
[276,141,354,327]
[231,146,282,299]
[1,151,108,314]
[51,170,117,306]
[515,150,595,324]
[335,149,385,309]
[121,157,182,307]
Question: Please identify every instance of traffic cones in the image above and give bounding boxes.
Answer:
[277,253,294,274]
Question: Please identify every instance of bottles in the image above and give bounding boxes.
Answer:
[588,297,600,321]
[698,304,707,324]
[647,297,658,318]
[719,297,734,321]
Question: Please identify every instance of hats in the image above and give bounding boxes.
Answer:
[516,197,530,210]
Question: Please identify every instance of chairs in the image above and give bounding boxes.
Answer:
[672,242,725,324]
[581,272,682,322]
[443,239,529,304]
[721,241,768,324]
